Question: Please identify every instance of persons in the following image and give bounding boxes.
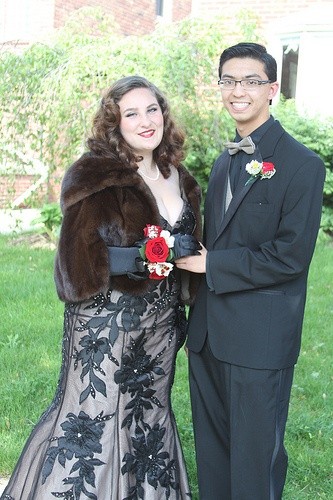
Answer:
[177,43,326,500]
[2,76,203,500]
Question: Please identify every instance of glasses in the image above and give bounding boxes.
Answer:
[218,78,271,91]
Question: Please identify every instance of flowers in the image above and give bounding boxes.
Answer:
[244,159,277,180]
[138,224,176,281]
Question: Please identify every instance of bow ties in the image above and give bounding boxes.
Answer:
[223,136,256,156]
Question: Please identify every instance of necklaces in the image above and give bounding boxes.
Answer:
[135,163,161,180]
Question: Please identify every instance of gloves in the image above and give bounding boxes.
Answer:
[105,233,203,276]
